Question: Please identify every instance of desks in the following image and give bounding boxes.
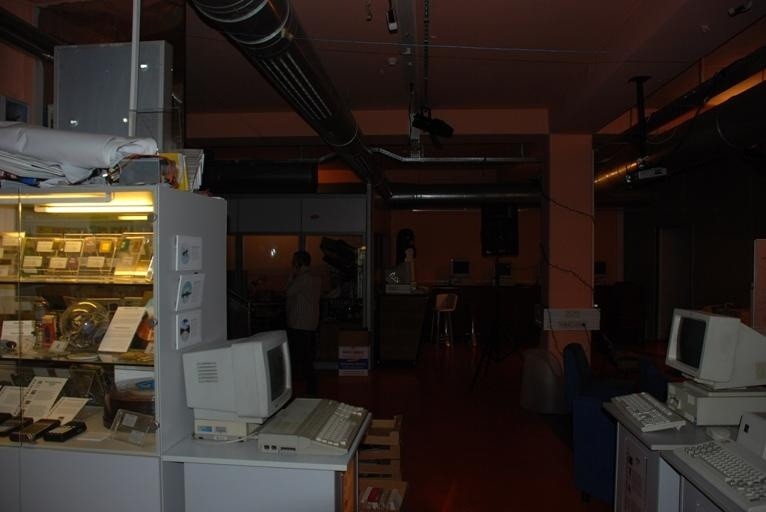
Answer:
[602,402,766,512]
[163,412,373,511]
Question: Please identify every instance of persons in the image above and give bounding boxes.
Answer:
[394,227,419,283]
[256,250,326,400]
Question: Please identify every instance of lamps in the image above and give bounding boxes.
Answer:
[411,105,455,141]
[627,74,717,185]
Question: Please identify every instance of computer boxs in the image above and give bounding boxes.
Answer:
[666,382,766,426]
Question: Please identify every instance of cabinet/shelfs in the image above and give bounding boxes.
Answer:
[0,182,228,512]
[226,196,369,235]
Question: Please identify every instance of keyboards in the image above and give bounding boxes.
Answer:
[613,391,685,431]
[258,398,369,455]
[673,438,766,512]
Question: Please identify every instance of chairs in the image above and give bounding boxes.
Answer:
[562,343,636,417]
[431,293,459,350]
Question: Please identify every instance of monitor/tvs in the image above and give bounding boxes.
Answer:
[595,261,607,275]
[664,308,766,397]
[494,262,512,277]
[450,259,470,276]
[183,330,292,425]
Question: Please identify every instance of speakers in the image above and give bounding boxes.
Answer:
[481,201,518,257]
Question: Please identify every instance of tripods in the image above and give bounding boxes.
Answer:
[467,258,524,389]
